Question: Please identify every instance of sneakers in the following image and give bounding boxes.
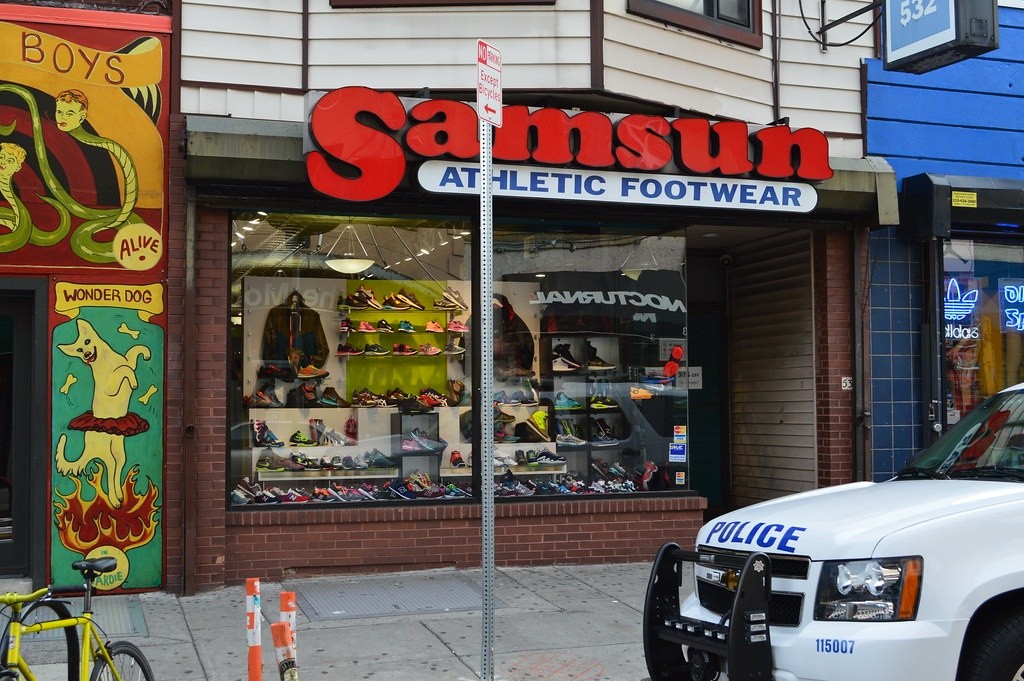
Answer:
[337,294,368,310]
[392,344,417,355]
[410,428,434,450]
[448,322,469,332]
[425,321,444,332]
[450,451,465,467]
[493,366,539,404]
[417,344,441,355]
[358,321,377,332]
[340,318,356,332]
[250,418,357,447]
[444,287,468,310]
[553,336,616,371]
[402,440,421,450]
[256,449,397,472]
[467,451,472,467]
[664,346,682,377]
[354,284,382,310]
[555,392,621,447]
[365,344,390,355]
[337,343,364,355]
[231,471,472,505]
[377,320,394,333]
[397,289,425,310]
[495,407,548,442]
[433,300,456,310]
[493,447,675,497]
[398,321,416,332]
[383,293,410,311]
[244,364,466,413]
[443,344,465,354]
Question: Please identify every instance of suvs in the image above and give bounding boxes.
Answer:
[642,383,1024,681]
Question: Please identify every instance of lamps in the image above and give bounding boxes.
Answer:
[421,236,430,255]
[403,248,412,261]
[460,220,471,235]
[437,228,449,246]
[384,260,391,270]
[414,241,423,257]
[324,216,375,274]
[452,223,462,239]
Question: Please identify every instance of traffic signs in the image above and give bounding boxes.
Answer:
[477,39,502,128]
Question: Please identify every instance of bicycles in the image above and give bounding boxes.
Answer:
[0,556,155,681]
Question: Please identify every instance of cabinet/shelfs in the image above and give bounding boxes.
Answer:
[539,332,654,480]
[240,275,568,484]
